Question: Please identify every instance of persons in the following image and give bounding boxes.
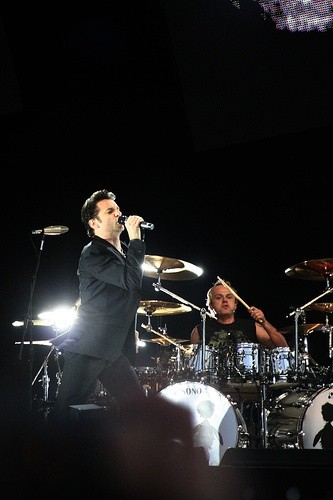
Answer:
[51,189,149,420]
[190,280,289,348]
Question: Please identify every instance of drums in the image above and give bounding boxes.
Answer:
[267,382,333,450]
[155,381,239,466]
[270,346,306,381]
[168,344,221,373]
[220,342,271,376]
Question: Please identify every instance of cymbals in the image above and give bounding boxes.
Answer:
[290,303,333,313]
[285,258,333,281]
[141,255,204,280]
[137,301,192,316]
[141,337,190,346]
[276,324,330,335]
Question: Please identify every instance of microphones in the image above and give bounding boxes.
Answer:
[118,215,154,230]
[31,225,69,235]
